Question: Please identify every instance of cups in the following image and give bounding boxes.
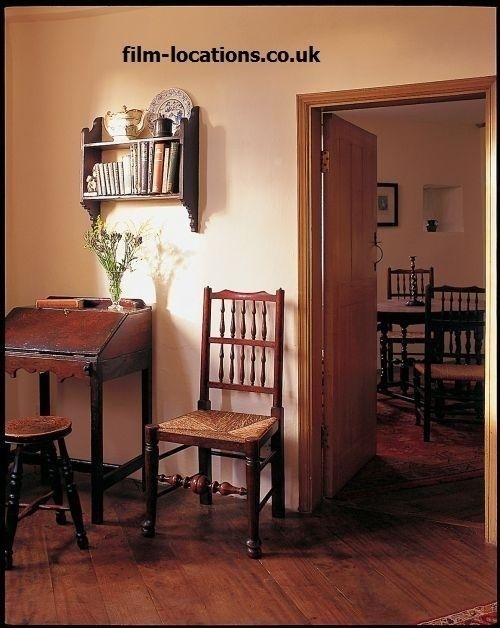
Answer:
[153,115,174,138]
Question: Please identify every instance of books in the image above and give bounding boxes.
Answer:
[84,141,180,196]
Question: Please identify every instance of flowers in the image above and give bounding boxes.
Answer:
[81,213,148,301]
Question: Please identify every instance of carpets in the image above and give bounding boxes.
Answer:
[418,598,497,628]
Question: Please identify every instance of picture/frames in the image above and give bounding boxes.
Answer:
[372,181,400,229]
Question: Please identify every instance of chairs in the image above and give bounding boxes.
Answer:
[134,280,287,560]
[375,265,488,442]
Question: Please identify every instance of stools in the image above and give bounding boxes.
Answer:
[3,415,91,572]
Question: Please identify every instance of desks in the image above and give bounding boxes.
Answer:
[2,293,152,525]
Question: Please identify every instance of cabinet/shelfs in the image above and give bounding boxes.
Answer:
[77,105,203,234]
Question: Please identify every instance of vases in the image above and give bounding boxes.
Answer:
[106,268,124,311]
